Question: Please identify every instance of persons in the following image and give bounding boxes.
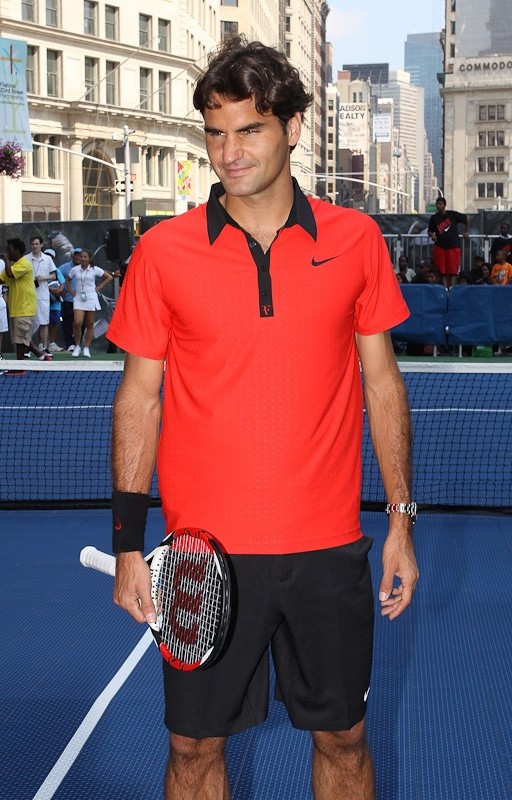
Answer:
[390,224,512,358]
[428,197,470,288]
[0,235,132,377]
[109,33,420,800]
[65,249,113,358]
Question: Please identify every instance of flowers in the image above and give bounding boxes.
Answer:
[0,137,25,179]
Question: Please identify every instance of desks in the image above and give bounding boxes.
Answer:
[388,284,512,360]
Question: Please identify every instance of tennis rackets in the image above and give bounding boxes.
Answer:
[80,527,235,674]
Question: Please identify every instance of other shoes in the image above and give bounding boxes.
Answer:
[24,349,34,358]
[4,369,27,376]
[0,355,9,374]
[49,344,63,352]
[43,348,54,358]
[83,346,92,357]
[67,344,75,351]
[71,346,83,358]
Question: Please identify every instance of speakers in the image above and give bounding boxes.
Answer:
[106,228,133,263]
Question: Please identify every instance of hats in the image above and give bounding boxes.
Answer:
[43,249,56,258]
[74,248,84,253]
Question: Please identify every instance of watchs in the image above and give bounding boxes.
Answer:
[384,501,418,525]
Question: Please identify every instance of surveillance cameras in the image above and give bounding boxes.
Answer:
[112,136,119,141]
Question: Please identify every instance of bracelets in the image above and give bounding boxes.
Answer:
[111,271,115,276]
